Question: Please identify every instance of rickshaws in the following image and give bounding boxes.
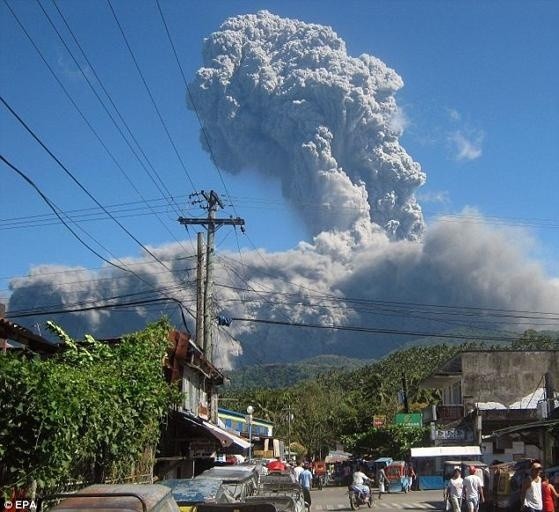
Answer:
[311,462,328,490]
[375,461,410,493]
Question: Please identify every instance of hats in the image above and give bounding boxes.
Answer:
[532,462,543,470]
[469,466,476,473]
[454,466,461,472]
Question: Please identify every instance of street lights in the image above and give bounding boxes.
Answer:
[288,413,294,462]
[247,405,255,464]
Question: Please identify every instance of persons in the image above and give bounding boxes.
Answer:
[407,466,416,492]
[376,464,390,500]
[462,466,486,512]
[352,466,375,503]
[539,472,559,512]
[400,474,410,493]
[265,457,335,492]
[521,462,543,512]
[444,468,466,512]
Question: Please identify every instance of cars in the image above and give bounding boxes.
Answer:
[37,454,311,512]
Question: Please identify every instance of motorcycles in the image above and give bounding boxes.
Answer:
[348,479,375,511]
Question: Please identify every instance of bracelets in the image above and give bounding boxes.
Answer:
[521,506,524,507]
